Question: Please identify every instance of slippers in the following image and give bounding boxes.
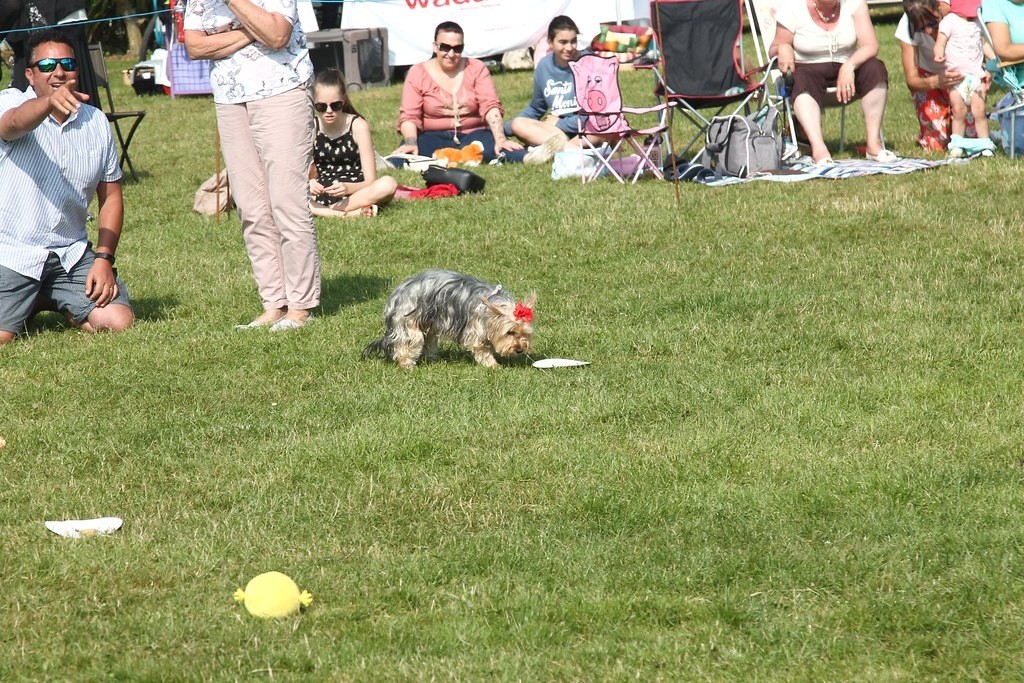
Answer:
[671,164,704,180]
[696,169,723,185]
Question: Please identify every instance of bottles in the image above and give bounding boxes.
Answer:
[642,134,660,171]
[725,87,743,96]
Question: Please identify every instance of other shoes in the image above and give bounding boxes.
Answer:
[522,133,566,167]
[269,312,318,333]
[866,144,898,162]
[360,204,378,217]
[236,310,286,330]
[816,157,836,167]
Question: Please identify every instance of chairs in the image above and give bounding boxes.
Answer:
[632,0,798,177]
[744,0,887,160]
[87,41,147,184]
[568,54,677,188]
[976,6,1024,161]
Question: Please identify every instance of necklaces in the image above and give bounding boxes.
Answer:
[814,2,839,22]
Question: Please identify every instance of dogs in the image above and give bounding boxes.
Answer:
[359,267,538,371]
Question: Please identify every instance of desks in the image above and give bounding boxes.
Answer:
[306,26,389,100]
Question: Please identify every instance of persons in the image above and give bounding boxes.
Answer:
[504,15,608,149]
[184,0,322,332]
[894,0,992,152]
[769,0,897,167]
[933,0,994,158]
[389,21,565,170]
[0,32,137,344]
[982,0,1024,84]
[309,69,398,218]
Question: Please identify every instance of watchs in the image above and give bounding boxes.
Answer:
[95,253,115,265]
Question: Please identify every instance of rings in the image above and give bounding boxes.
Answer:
[952,78,954,81]
[846,85,851,89]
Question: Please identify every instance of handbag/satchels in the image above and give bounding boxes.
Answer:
[420,164,486,194]
[704,104,783,178]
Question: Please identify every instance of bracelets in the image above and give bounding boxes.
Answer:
[225,0,232,5]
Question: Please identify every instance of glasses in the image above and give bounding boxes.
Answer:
[436,41,464,53]
[27,57,79,72]
[314,100,345,112]
[915,19,938,32]
